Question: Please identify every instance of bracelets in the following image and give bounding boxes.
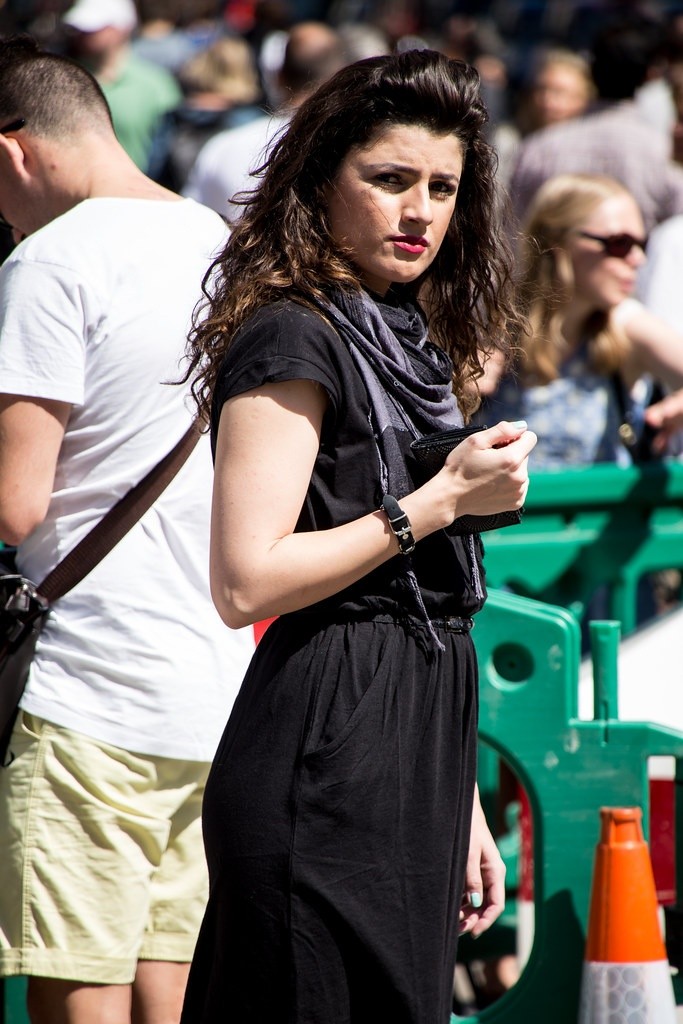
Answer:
[380,495,415,556]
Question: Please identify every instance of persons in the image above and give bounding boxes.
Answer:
[0,0,683,318]
[459,175,683,660]
[0,52,257,1024]
[160,51,535,1024]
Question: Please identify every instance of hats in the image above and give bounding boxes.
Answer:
[60,0,137,37]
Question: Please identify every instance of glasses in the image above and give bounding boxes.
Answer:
[581,232,650,259]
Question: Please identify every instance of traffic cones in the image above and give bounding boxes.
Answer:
[574,805,679,1023]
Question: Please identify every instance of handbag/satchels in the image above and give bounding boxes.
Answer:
[0,546,50,768]
